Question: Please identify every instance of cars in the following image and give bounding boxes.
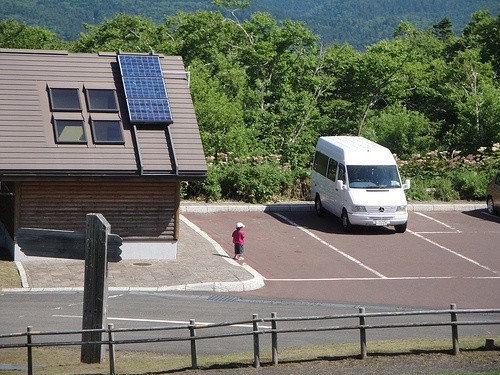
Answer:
[485,173,500,213]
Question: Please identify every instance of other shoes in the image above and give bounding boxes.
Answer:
[234,257,238,259]
[238,257,244,261]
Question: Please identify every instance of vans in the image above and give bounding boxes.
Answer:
[310,134,409,232]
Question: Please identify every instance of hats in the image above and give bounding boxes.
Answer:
[236,222,245,229]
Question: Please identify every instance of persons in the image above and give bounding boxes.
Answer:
[232,222,245,261]
